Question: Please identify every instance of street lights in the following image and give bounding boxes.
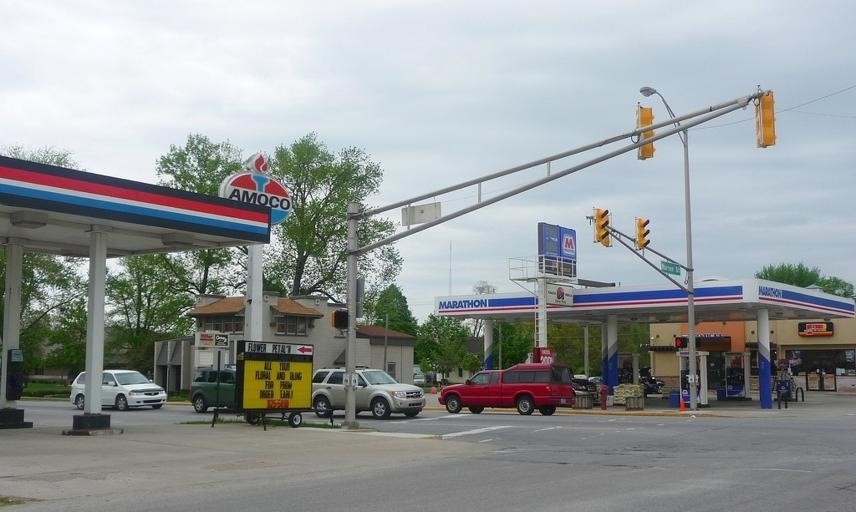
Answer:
[638,85,698,411]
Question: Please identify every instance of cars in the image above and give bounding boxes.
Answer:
[70,370,167,412]
[570,374,665,394]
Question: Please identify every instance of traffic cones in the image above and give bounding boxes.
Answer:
[679,398,687,411]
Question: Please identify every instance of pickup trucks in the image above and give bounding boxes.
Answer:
[437,362,577,417]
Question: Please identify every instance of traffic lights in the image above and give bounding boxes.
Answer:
[675,336,687,349]
[594,208,611,248]
[332,310,348,329]
[635,217,651,251]
[637,107,657,160]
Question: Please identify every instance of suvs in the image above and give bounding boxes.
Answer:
[311,366,426,420]
[190,368,237,412]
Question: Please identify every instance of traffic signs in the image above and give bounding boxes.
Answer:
[661,261,681,275]
